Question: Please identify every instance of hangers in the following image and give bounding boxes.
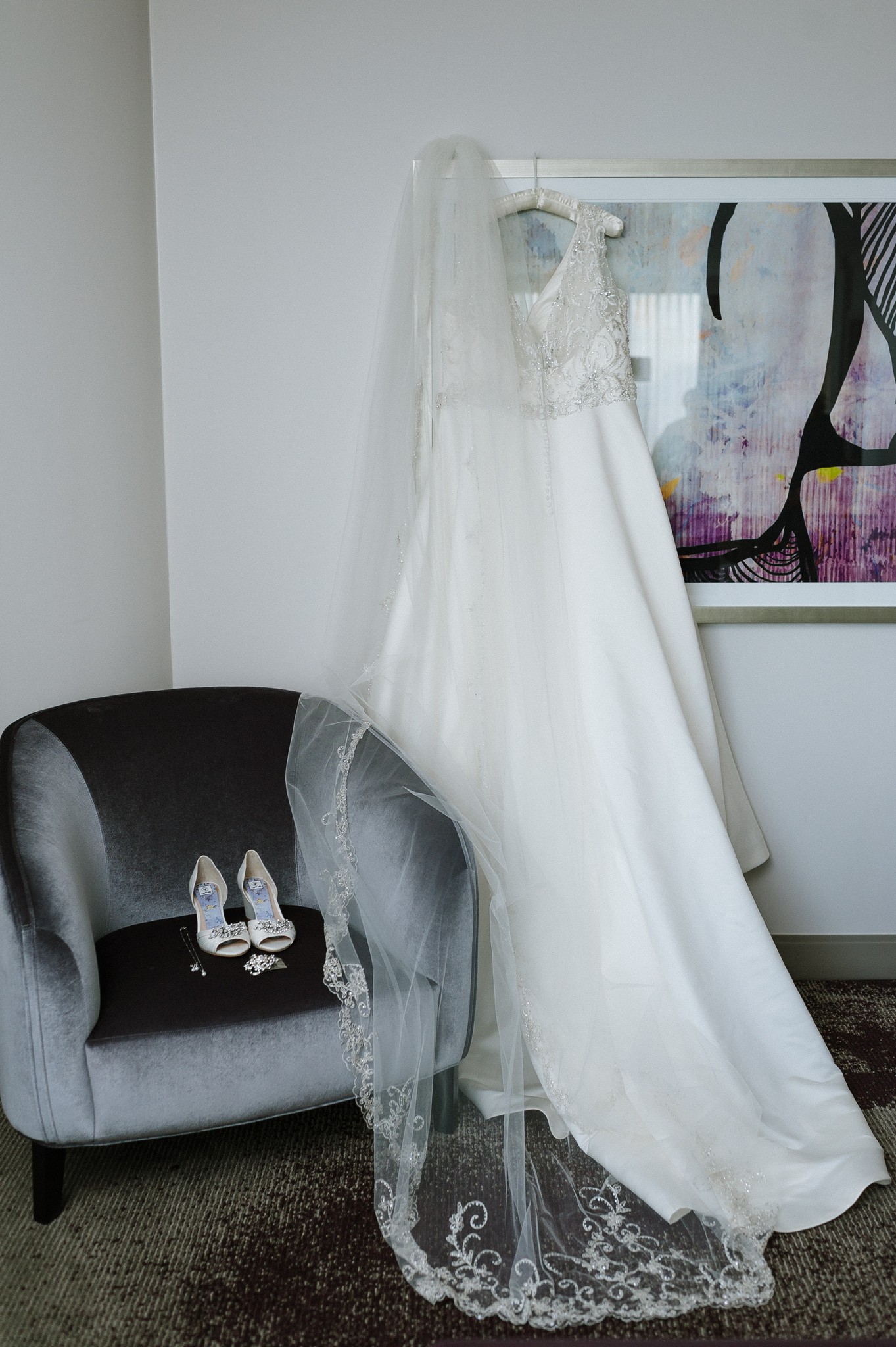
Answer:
[472,153,625,237]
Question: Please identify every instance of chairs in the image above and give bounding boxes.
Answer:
[0,684,485,1216]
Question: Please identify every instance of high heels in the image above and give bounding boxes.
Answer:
[237,850,297,953]
[189,854,251,959]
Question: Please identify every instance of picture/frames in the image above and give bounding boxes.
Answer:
[404,146,896,625]
